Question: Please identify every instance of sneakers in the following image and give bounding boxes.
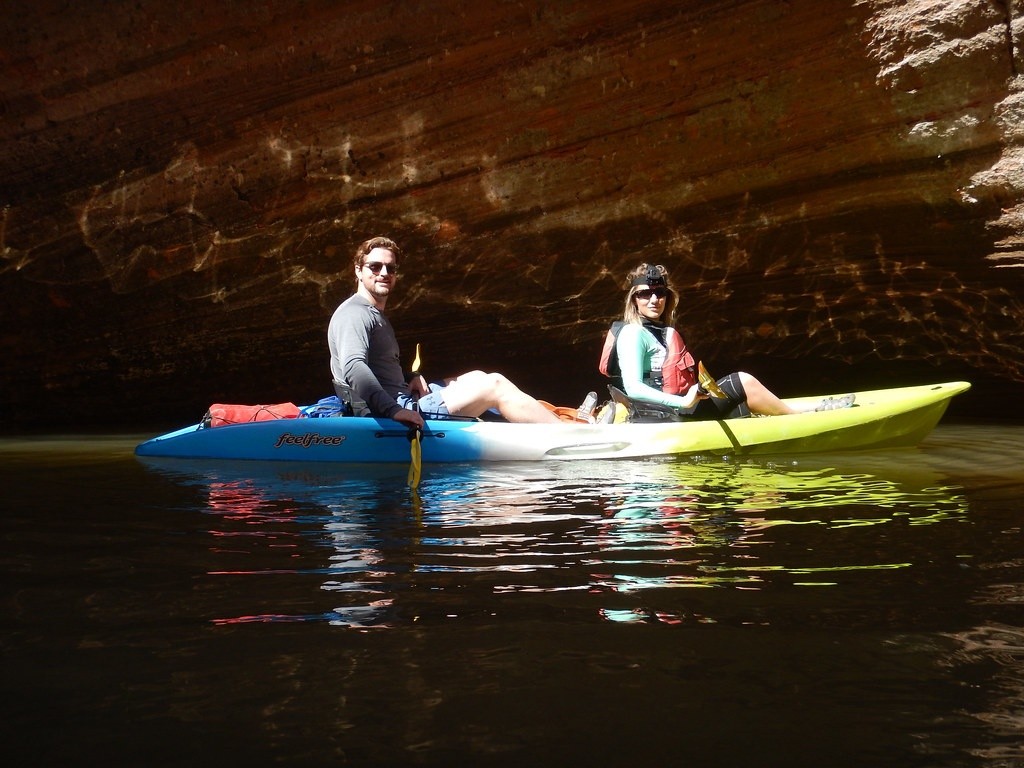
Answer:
[814,394,855,412]
[595,402,616,423]
[575,392,598,414]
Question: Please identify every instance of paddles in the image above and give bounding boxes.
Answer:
[408,342,422,492]
[696,359,728,402]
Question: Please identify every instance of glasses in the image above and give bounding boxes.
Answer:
[635,287,666,299]
[362,262,398,273]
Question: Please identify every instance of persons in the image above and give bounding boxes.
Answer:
[615,264,855,421]
[327,237,616,429]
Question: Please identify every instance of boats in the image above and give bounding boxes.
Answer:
[130,377,978,462]
